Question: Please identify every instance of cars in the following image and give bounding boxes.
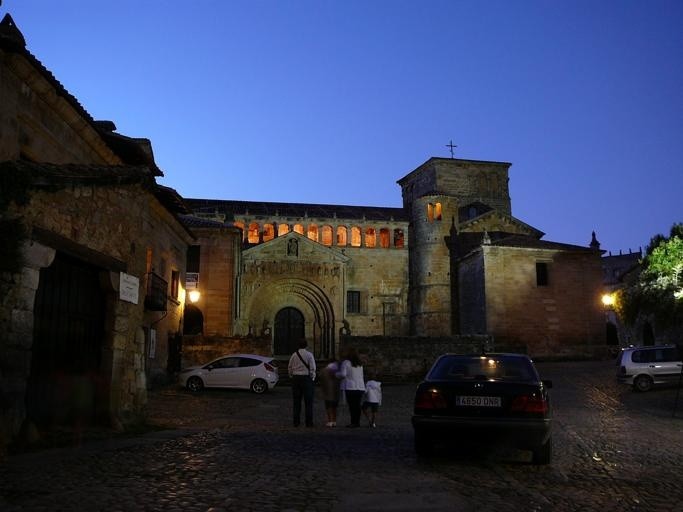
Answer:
[178,353,280,394]
[412,353,552,464]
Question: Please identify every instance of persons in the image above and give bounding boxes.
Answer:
[361,378,383,429]
[334,351,366,428]
[318,357,341,428]
[288,340,317,428]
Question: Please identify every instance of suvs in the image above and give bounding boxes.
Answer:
[615,345,683,393]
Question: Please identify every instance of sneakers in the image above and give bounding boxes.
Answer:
[368,421,376,428]
[326,422,336,427]
[345,424,360,428]
